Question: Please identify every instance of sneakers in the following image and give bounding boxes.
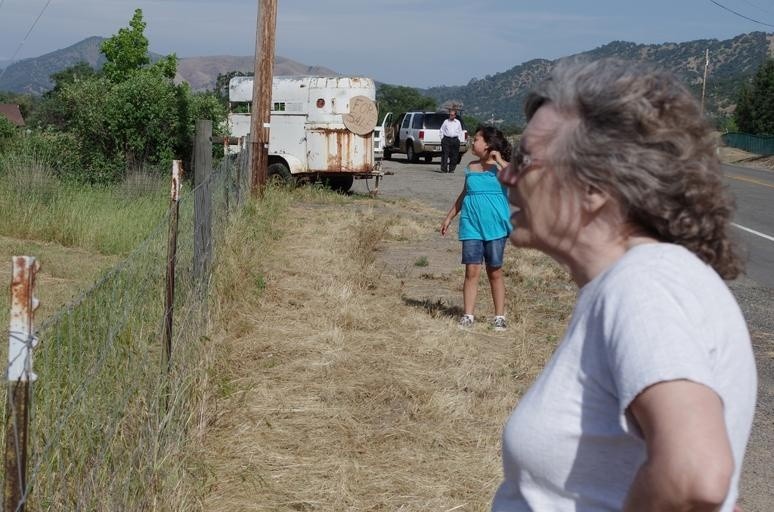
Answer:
[495,316,507,331]
[458,314,474,329]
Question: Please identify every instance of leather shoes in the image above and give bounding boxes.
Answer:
[440,170,455,173]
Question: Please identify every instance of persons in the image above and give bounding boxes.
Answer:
[439,109,463,174]
[489,55,759,512]
[434,125,513,333]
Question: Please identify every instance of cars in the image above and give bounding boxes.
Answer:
[379,111,471,164]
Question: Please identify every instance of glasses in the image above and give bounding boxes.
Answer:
[510,141,565,175]
